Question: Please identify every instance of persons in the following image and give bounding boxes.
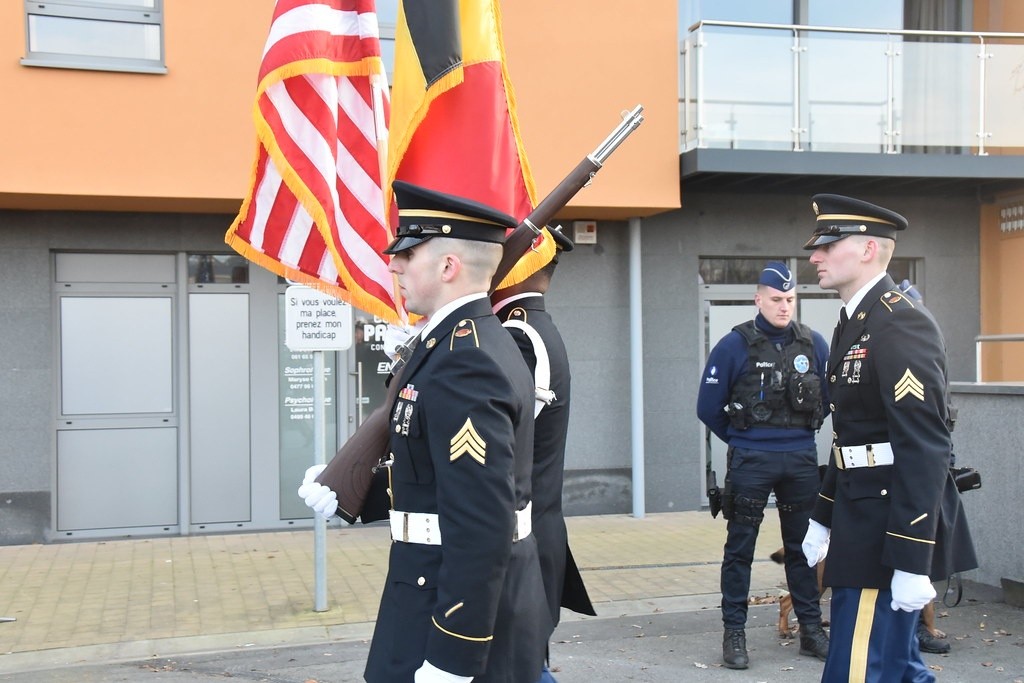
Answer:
[802,192,978,683]
[696,261,830,669]
[292,176,555,683]
[487,223,597,683]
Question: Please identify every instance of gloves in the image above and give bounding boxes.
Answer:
[298,465,339,520]
[802,518,831,568]
[414,659,474,683]
[891,568,936,612]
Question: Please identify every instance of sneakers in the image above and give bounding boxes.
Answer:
[723,629,749,669]
[799,623,830,661]
[915,621,950,653]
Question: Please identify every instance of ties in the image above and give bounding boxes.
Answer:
[838,307,849,340]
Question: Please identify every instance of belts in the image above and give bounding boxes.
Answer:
[389,500,532,547]
[832,442,894,470]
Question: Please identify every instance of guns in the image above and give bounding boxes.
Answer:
[309,98,652,527]
[704,469,720,520]
[949,464,983,494]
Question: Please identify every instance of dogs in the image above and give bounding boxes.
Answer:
[770,547,947,639]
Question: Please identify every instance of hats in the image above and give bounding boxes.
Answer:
[546,224,575,264]
[381,179,518,255]
[759,261,795,291]
[354,321,368,329]
[803,193,908,250]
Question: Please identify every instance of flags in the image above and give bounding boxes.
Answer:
[223,0,400,327]
[388,0,557,323]
